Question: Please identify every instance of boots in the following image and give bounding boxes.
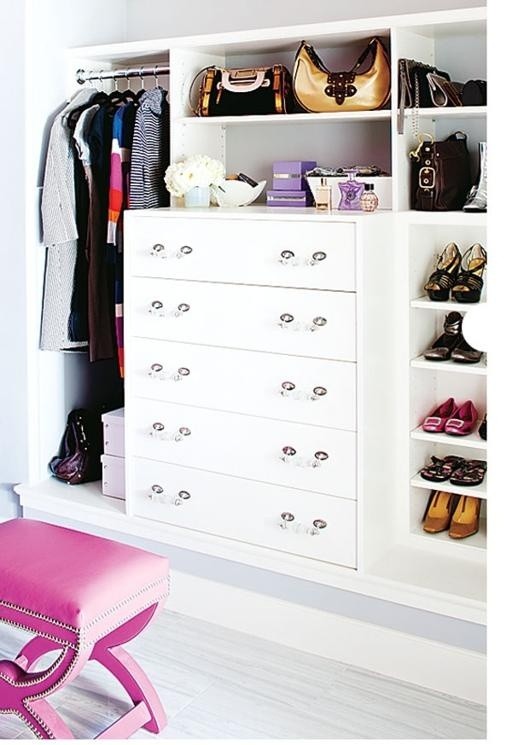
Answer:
[463,142,487,212]
[451,339,481,363]
[424,312,462,360]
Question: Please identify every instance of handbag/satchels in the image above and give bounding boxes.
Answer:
[291,40,391,112]
[199,64,292,115]
[53,403,112,485]
[399,59,487,109]
[411,139,471,211]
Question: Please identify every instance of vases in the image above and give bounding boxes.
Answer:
[183,187,210,207]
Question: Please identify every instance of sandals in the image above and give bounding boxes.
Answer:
[424,242,461,301]
[452,243,487,303]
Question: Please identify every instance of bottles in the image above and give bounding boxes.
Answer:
[314,177,332,206]
[338,170,364,210]
[359,184,379,211]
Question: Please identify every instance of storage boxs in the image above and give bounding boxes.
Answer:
[101,404,125,457]
[271,160,317,190]
[99,454,124,499]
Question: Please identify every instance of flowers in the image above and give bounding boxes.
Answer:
[163,153,226,198]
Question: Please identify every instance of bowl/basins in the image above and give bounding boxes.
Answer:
[208,179,267,209]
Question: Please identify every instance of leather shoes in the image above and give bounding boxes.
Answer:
[423,398,458,432]
[449,495,481,539]
[424,490,456,534]
[445,401,478,435]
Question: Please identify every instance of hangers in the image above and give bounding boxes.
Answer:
[61,63,165,130]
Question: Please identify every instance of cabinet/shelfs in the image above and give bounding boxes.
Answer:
[394,7,489,605]
[125,208,394,581]
[171,19,393,212]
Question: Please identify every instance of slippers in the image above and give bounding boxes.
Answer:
[420,456,465,482]
[450,460,487,486]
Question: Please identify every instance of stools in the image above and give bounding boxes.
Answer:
[0,515,169,739]
